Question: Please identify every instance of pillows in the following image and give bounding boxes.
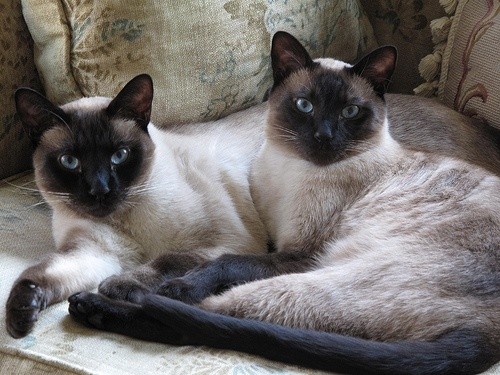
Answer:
[20,0,381,134]
[412,0,500,132]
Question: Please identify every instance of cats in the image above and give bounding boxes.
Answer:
[6,73,500,340]
[68,30,500,375]
